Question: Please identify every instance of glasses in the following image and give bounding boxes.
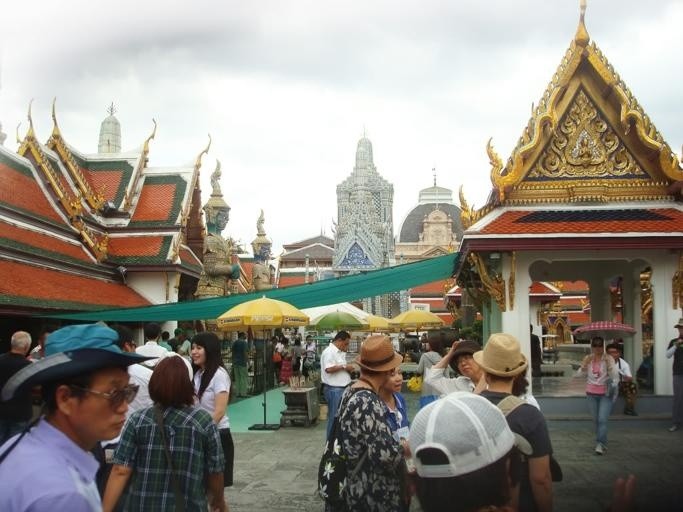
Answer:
[590,343,602,348]
[68,384,140,404]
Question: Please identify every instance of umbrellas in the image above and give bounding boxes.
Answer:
[363,316,400,334]
[216,294,309,426]
[393,307,444,335]
[305,310,370,333]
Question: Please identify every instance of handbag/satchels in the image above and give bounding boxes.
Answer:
[303,358,313,370]
[317,450,349,504]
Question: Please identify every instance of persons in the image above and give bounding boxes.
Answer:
[251,243,277,339]
[579,138,592,168]
[193,208,239,364]
[666,318,683,432]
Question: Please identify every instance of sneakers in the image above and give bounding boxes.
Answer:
[595,442,605,454]
[623,407,638,416]
[667,424,678,431]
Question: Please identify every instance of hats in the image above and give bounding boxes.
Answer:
[448,340,482,376]
[402,390,533,478]
[1,321,158,402]
[673,317,683,327]
[352,334,403,372]
[472,332,527,377]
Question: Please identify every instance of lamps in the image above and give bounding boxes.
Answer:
[100,200,116,214]
